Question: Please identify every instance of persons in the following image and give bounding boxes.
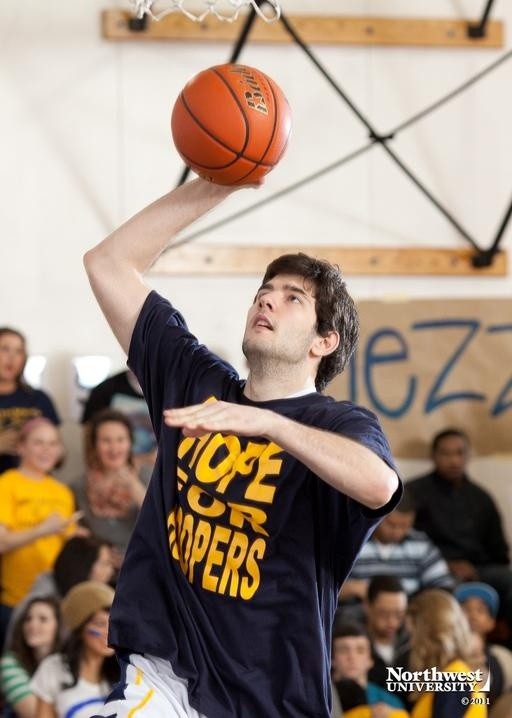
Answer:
[1,325,64,471]
[80,368,159,471]
[2,410,511,717]
[82,170,406,716]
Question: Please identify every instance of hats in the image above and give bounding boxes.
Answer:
[59,579,118,642]
[450,580,501,622]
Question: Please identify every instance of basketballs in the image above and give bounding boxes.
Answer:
[172,65,292,186]
